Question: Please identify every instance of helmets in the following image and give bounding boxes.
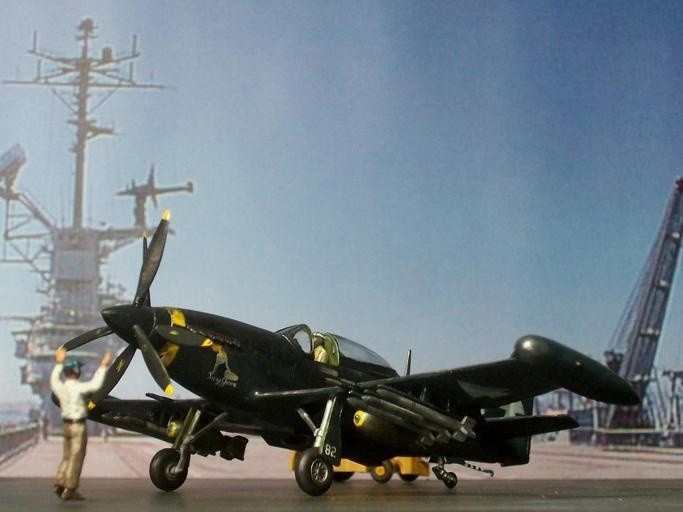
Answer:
[63,355,86,375]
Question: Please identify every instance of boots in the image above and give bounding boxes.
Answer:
[54,484,85,501]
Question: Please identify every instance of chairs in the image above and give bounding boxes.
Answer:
[323,338,335,365]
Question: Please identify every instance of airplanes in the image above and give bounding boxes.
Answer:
[51,209,641,496]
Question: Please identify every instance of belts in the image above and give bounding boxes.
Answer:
[62,418,87,423]
